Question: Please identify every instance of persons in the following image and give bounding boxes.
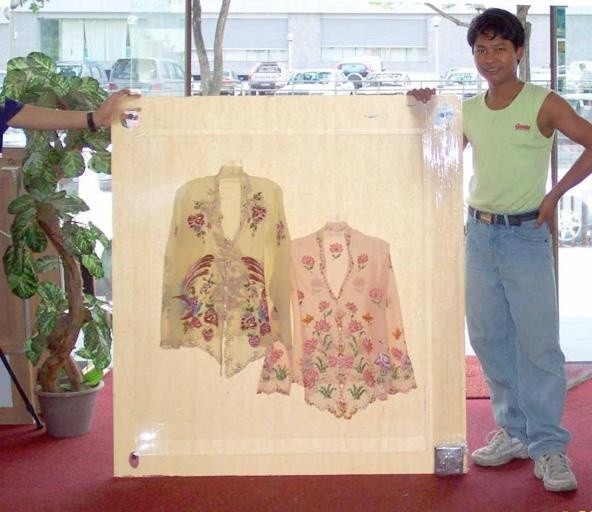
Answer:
[0,88,143,155]
[406,8,591,494]
[574,62,591,108]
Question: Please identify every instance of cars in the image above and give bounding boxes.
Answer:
[190,74,203,95]
[358,72,415,95]
[437,60,592,247]
[221,68,243,95]
[274,67,355,96]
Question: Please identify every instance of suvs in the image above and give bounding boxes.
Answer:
[249,60,286,95]
[336,61,370,88]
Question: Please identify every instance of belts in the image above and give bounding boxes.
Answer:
[467,205,540,226]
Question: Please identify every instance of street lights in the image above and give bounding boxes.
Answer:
[432,16,442,81]
[125,13,140,57]
[286,32,294,71]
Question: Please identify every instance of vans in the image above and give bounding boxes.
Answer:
[110,57,194,97]
[56,61,110,96]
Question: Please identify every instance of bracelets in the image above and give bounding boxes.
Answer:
[87,111,99,132]
[79,111,86,130]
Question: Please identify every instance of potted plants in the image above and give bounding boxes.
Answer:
[0,49,113,438]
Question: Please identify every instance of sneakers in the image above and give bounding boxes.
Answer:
[534,451,577,493]
[471,428,530,467]
